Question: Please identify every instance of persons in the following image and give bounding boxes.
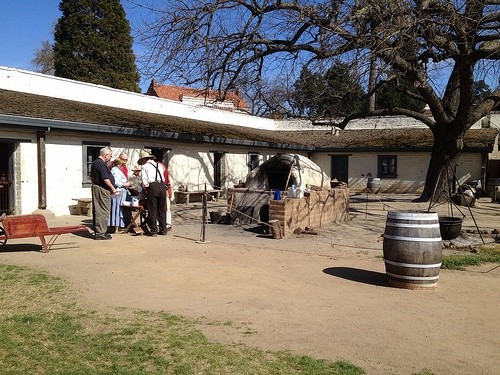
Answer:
[129,165,142,182]
[137,152,167,237]
[107,156,131,234]
[90,146,117,240]
[0,173,9,216]
[233,180,245,188]
[108,152,128,181]
[154,156,172,231]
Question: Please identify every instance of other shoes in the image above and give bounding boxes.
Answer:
[94,234,112,240]
[146,231,156,237]
[167,226,171,230]
[158,231,167,235]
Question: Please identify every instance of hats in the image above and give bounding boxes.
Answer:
[137,152,154,165]
[132,166,141,171]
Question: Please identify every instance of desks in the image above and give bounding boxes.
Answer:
[72,198,93,218]
[174,189,224,205]
[120,205,144,237]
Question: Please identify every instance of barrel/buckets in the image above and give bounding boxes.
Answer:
[366,178,381,194]
[210,210,222,224]
[383,209,442,287]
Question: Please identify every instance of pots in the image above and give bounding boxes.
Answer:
[438,216,463,240]
